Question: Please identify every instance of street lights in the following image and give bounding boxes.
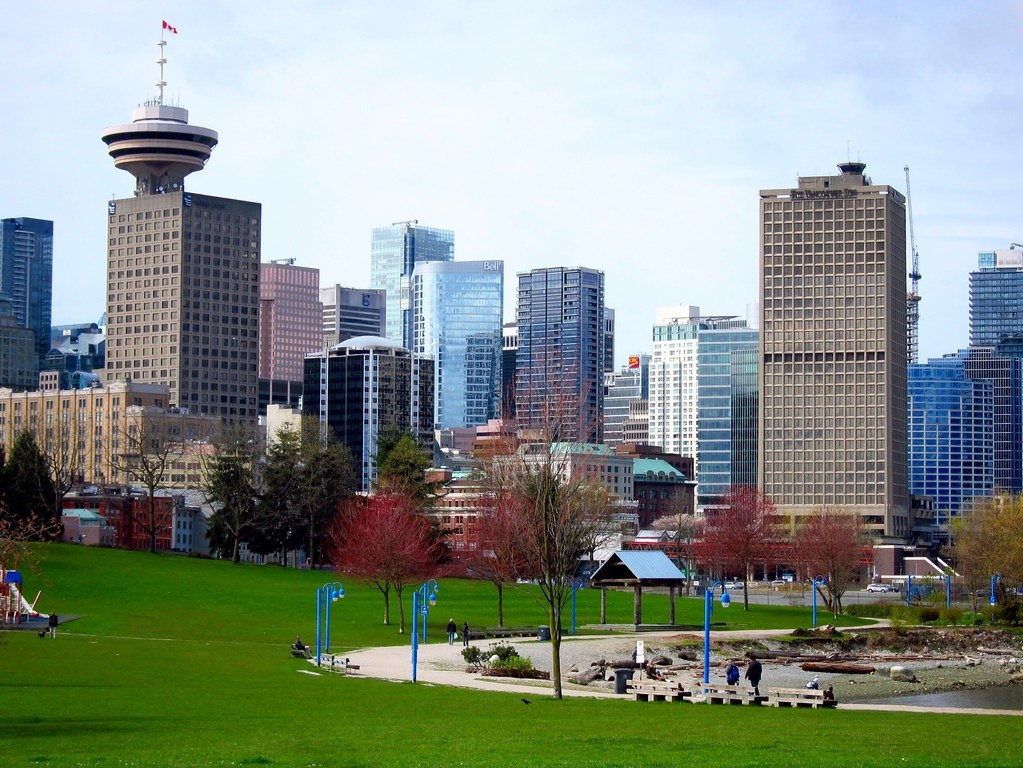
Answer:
[424,579,440,643]
[705,582,731,695]
[412,585,437,683]
[813,575,826,626]
[908,572,915,605]
[572,576,585,635]
[316,583,339,667]
[325,582,345,653]
[990,572,1001,605]
[940,572,950,606]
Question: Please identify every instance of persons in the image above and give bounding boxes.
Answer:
[724,658,740,694]
[744,653,762,696]
[805,675,835,700]
[447,619,456,645]
[49,611,58,640]
[462,622,469,646]
[645,661,666,682]
[725,576,727,582]
[632,647,637,670]
[295,636,313,659]
[78,534,82,545]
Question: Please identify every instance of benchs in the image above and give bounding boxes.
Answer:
[290,642,360,676]
[462,626,539,640]
[625,678,838,710]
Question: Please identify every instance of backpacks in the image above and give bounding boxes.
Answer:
[728,666,739,681]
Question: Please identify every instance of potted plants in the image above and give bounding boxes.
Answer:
[459,641,551,681]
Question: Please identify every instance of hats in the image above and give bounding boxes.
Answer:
[813,678,818,683]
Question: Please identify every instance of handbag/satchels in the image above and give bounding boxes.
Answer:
[454,633,458,639]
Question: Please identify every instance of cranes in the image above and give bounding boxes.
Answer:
[392,219,418,229]
[905,164,921,363]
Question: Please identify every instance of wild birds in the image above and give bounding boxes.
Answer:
[521,699,532,704]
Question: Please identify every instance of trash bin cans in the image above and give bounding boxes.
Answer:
[537,626,551,641]
[613,668,635,694]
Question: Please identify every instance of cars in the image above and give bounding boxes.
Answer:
[884,586,898,592]
[867,584,888,593]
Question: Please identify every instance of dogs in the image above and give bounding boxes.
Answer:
[37,630,45,639]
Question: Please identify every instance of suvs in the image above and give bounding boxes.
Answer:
[720,582,744,589]
